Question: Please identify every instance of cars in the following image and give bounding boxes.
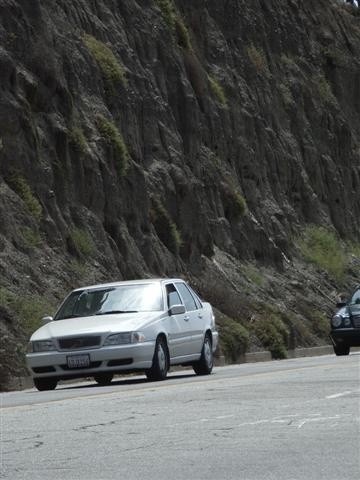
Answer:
[329,286,360,355]
[26,278,220,393]
